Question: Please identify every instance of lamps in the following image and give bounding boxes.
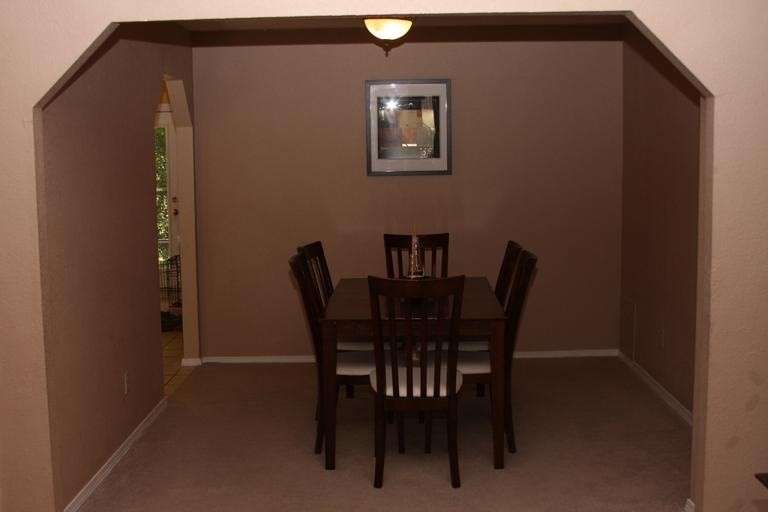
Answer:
[362,14,414,56]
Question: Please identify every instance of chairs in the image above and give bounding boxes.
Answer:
[288,239,407,473]
[381,232,451,278]
[421,237,537,470]
[352,272,469,488]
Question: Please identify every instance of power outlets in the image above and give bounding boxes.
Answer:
[123,371,130,395]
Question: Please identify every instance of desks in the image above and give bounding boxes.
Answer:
[320,273,513,470]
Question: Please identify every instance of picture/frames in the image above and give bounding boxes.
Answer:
[365,79,452,178]
